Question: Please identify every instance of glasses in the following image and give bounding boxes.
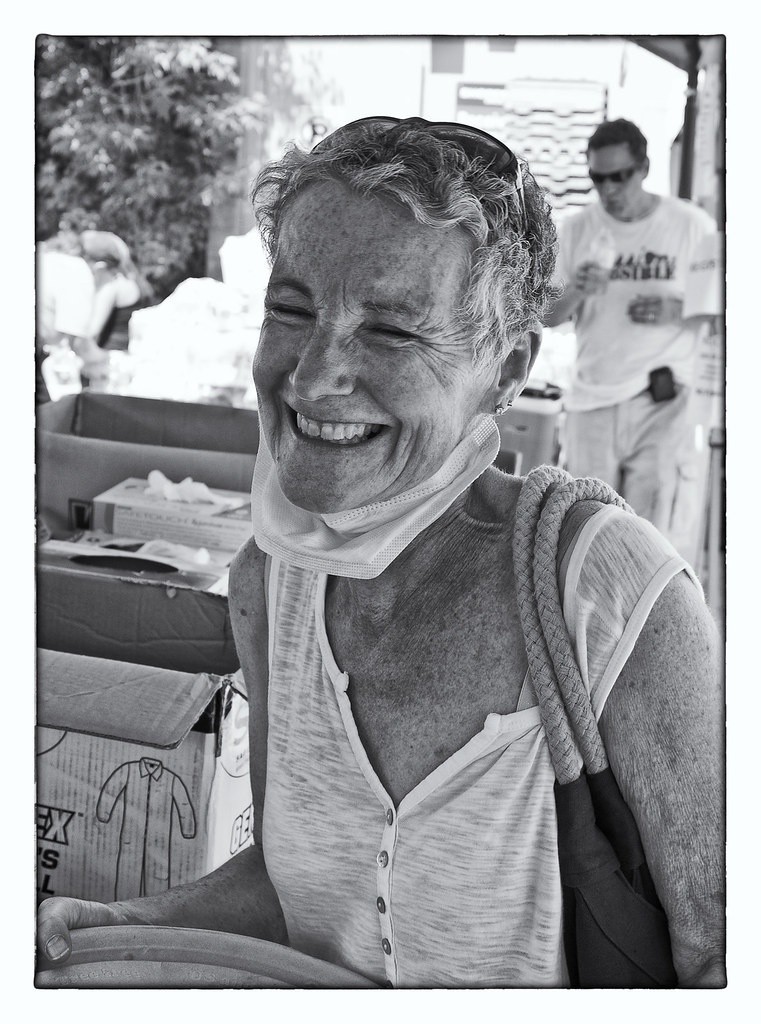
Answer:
[587,158,643,185]
[306,113,533,254]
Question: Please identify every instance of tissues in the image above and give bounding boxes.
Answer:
[91,469,256,563]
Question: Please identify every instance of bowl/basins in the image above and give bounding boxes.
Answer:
[37,925,381,990]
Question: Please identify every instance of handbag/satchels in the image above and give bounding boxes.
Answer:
[513,464,682,989]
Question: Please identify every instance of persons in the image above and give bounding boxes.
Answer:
[34,115,724,989]
[36,195,108,405]
[539,117,724,535]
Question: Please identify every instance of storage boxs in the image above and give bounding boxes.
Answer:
[38,393,260,529]
[89,476,254,553]
[66,530,234,597]
[37,563,254,904]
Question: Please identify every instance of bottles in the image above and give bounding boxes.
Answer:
[585,224,616,295]
